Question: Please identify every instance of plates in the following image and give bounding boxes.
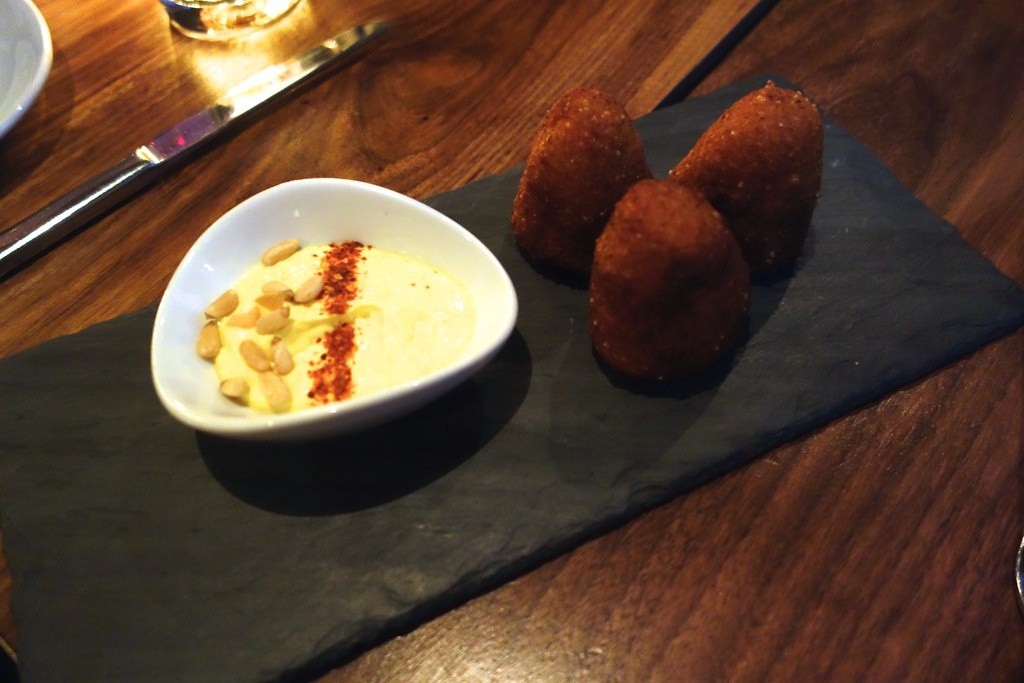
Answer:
[0,74,1024,683]
[0,1,53,135]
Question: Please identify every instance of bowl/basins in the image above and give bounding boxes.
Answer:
[148,178,521,439]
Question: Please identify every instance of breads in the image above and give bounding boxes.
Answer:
[512,80,825,386]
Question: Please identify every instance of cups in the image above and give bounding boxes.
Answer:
[158,0,300,40]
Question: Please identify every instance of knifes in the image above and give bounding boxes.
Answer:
[0,20,394,275]
